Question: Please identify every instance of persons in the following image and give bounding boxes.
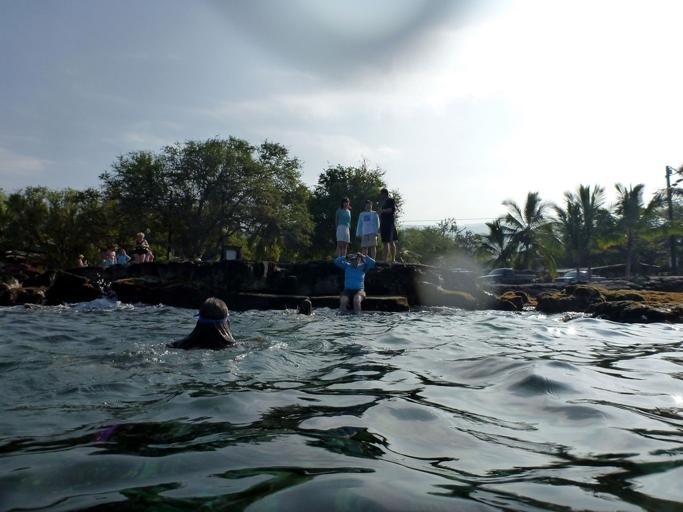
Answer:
[76,232,155,268]
[298,299,313,315]
[376,188,398,263]
[166,296,244,349]
[335,197,352,258]
[355,200,380,260]
[334,252,376,314]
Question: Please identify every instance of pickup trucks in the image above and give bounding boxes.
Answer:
[552,270,606,284]
[479,267,536,284]
